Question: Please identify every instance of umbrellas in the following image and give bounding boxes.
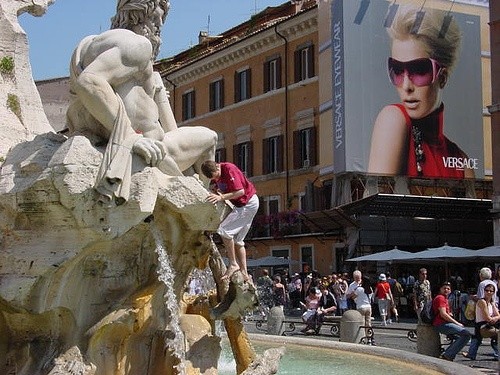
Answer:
[343,242,500,283]
[247,256,305,267]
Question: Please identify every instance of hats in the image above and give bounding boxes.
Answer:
[379,274,386,280]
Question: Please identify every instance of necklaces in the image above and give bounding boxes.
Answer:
[415,143,424,176]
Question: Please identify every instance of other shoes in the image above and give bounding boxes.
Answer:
[465,300,476,321]
[386,319,392,323]
[437,352,453,362]
[381,321,386,326]
[462,352,476,360]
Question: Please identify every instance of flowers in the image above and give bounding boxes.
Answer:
[254,209,304,239]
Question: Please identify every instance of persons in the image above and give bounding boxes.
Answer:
[431,281,470,362]
[249,266,406,339]
[461,267,500,360]
[412,267,432,335]
[66,0,219,204]
[368,9,475,179]
[201,161,259,281]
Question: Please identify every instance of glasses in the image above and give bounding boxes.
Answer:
[484,289,494,292]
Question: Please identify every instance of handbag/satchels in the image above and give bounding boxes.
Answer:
[381,282,392,300]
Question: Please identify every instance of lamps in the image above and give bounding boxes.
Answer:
[456,187,466,197]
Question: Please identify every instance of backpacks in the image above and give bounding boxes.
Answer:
[392,279,404,297]
[420,293,446,325]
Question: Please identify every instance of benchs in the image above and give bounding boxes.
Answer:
[324,316,342,322]
